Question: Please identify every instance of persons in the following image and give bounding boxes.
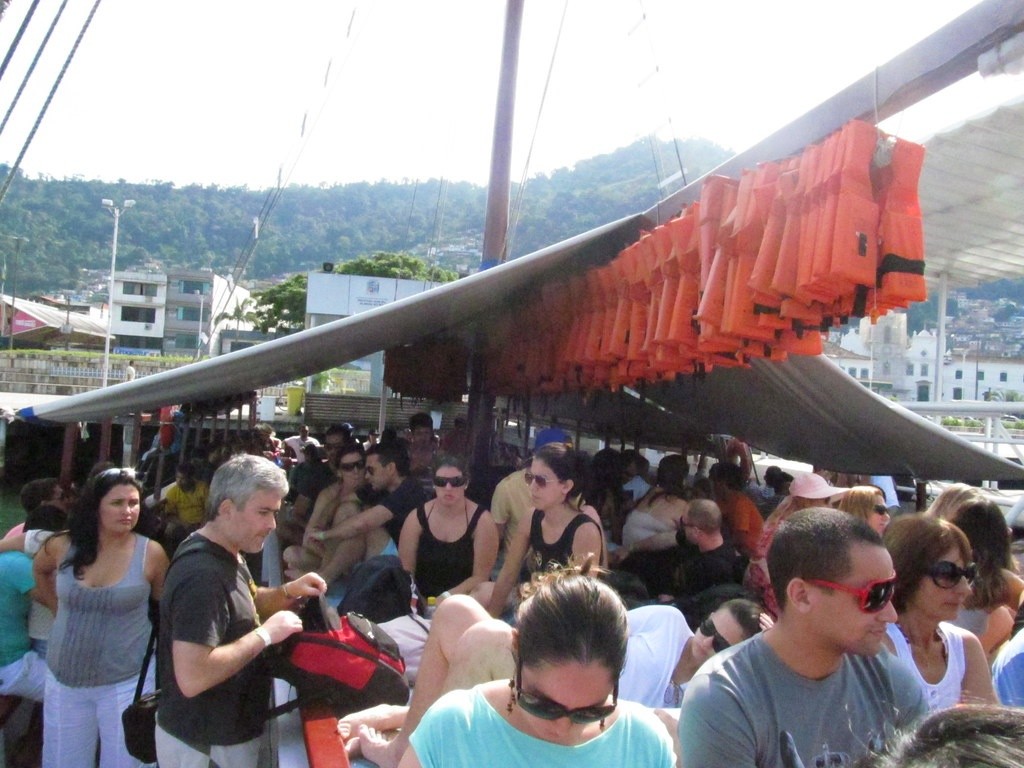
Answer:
[0,413,1024,768]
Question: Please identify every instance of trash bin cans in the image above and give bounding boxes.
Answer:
[287,386,303,415]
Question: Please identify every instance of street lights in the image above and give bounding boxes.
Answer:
[98,194,137,390]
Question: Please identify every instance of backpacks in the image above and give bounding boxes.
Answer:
[336,560,431,686]
[281,592,409,705]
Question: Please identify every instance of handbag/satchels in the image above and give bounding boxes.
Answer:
[293,493,313,517]
[122,689,161,763]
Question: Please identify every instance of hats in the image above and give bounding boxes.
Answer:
[342,423,354,430]
[369,429,380,436]
[789,472,851,502]
[535,429,567,448]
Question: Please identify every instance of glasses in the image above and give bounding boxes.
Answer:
[324,443,343,450]
[46,491,65,502]
[805,570,896,613]
[700,618,731,653]
[680,518,701,530]
[873,505,887,514]
[299,430,308,433]
[434,476,466,487]
[919,561,976,588]
[517,657,619,724]
[525,474,561,488]
[365,466,376,475]
[341,460,365,471]
[92,468,136,488]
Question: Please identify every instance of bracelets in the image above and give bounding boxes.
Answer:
[253,626,272,648]
[281,582,297,600]
